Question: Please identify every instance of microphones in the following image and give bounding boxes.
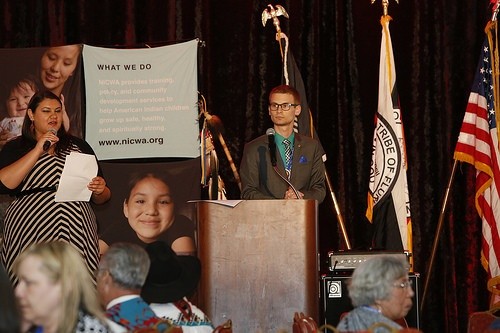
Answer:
[42,128,57,151]
[266,128,278,167]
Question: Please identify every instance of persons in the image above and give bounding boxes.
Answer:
[14,242,127,333]
[0,44,82,149]
[240,85,326,203]
[0,72,38,141]
[335,256,414,333]
[0,90,112,318]
[95,243,182,333]
[96,168,196,254]
[139,241,216,333]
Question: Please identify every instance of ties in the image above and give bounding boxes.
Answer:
[282,139,292,171]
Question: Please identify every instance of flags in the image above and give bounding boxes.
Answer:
[366,14,413,273]
[454,21,500,311]
[275,32,327,163]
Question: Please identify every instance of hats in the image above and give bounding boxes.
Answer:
[140,240,202,303]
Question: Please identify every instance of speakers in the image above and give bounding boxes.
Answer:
[320,274,420,333]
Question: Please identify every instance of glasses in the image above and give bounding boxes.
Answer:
[269,102,299,111]
[393,280,412,288]
